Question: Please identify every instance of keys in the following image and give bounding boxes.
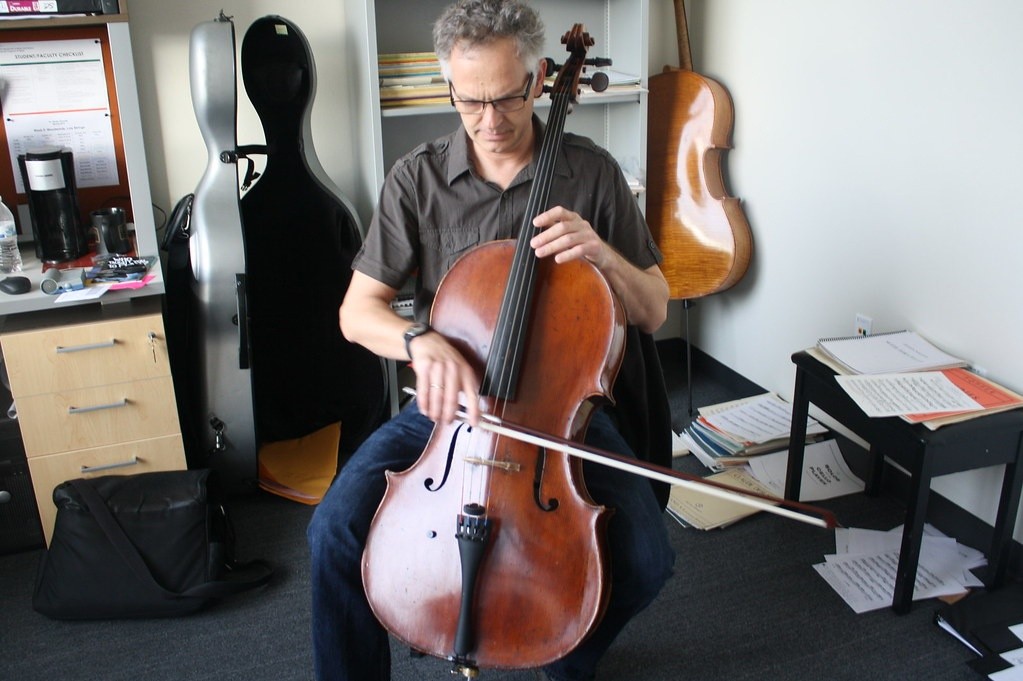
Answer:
[150,335,156,362]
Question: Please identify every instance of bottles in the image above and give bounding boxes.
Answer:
[0,196,24,274]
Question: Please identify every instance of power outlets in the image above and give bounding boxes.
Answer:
[853,314,873,336]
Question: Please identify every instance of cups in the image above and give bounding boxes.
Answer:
[90,207,132,254]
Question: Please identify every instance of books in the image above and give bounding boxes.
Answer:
[682,395,820,472]
[665,467,783,530]
[818,329,1023,431]
[546,71,642,96]
[934,584,1022,657]
[377,50,454,106]
[391,290,416,317]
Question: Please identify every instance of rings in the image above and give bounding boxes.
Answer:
[430,384,444,388]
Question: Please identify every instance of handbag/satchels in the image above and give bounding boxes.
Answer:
[33,468,278,621]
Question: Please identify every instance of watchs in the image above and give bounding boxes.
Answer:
[404,322,435,360]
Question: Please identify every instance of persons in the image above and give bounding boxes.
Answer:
[306,0,669,680]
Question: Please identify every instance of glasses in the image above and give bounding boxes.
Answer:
[448,71,534,114]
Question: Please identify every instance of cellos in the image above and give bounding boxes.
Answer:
[646,0,752,417]
[360,23,627,681]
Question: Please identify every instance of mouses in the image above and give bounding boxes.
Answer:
[0,276,31,295]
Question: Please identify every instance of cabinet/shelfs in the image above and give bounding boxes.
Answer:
[0,0,206,551]
[345,0,650,425]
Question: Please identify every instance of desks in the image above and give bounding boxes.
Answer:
[783,351,1023,617]
